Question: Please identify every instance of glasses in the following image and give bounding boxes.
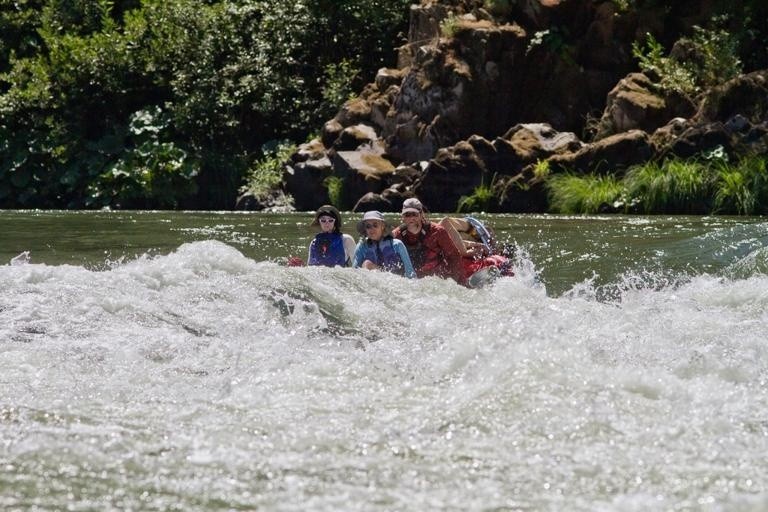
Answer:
[319,218,336,224]
[365,222,382,229]
[404,213,420,218]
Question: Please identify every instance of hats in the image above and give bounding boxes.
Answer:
[310,205,341,228]
[402,198,423,215]
[356,211,392,238]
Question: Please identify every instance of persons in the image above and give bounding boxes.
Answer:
[307,205,357,268]
[392,198,463,282]
[352,211,417,279]
